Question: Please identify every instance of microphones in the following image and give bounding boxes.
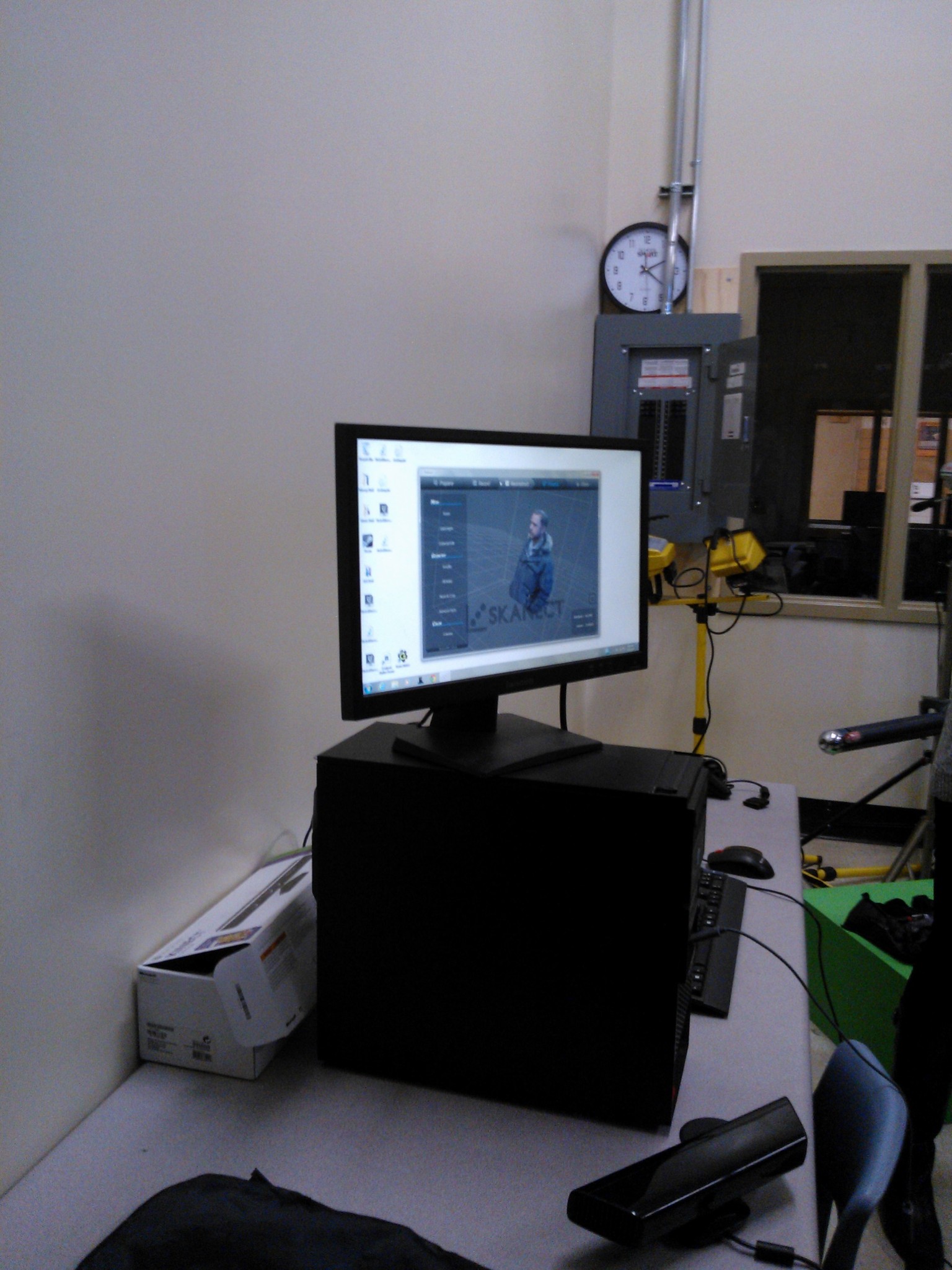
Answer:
[726,779,771,800]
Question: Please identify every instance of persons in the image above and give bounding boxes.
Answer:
[509,510,554,614]
[878,676,952,1270]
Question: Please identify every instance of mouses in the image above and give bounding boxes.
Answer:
[707,846,775,881]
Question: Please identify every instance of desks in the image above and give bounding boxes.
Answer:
[0,780,817,1270]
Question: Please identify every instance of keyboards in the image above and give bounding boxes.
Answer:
[688,867,748,1020]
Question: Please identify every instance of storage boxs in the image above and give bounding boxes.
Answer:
[137,845,318,1078]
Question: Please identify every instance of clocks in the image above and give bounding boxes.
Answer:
[600,222,689,315]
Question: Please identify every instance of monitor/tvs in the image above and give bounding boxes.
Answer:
[333,422,649,782]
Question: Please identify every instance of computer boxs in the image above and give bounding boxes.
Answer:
[311,720,712,1137]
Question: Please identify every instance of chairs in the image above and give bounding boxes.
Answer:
[811,1040,907,1270]
